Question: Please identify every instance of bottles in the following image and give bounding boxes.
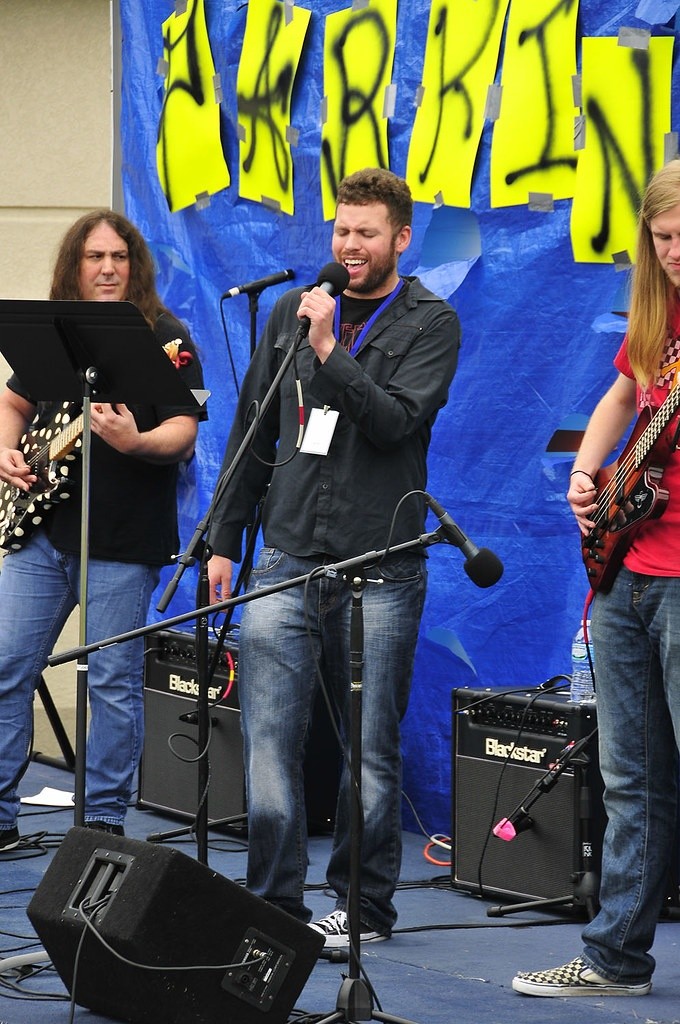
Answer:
[571,620,596,704]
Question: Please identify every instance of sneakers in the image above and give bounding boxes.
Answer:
[304,909,388,948]
[0,826,20,850]
[84,820,124,836]
[512,955,652,997]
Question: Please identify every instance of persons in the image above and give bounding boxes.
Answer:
[2,209,210,852]
[199,169,461,946]
[512,161,679,999]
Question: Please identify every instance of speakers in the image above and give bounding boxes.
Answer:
[26,825,325,1024]
[451,685,605,914]
[136,630,341,841]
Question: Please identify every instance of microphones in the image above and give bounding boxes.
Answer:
[422,491,504,587]
[178,714,219,728]
[222,268,295,299]
[492,817,534,838]
[294,262,350,338]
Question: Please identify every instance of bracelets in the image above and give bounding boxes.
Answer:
[570,470,593,482]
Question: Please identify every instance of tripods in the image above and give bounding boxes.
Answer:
[47,526,446,1024]
[487,726,600,923]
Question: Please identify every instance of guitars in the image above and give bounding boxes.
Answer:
[582,382,680,591]
[0,338,193,552]
